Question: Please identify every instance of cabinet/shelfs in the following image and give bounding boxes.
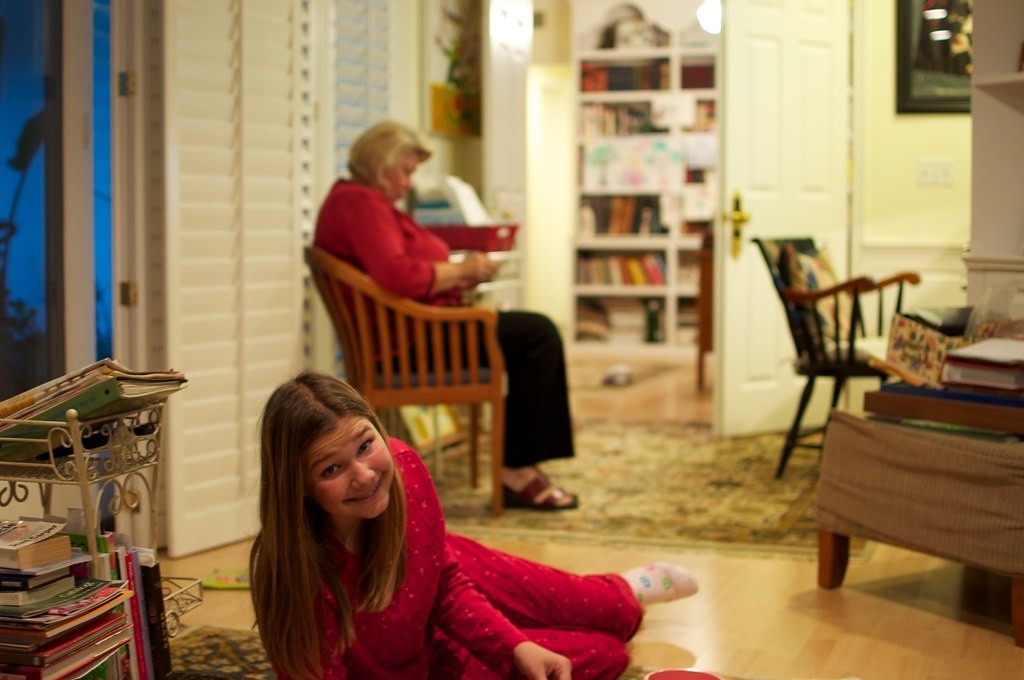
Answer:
[575,48,719,356]
[0,396,203,680]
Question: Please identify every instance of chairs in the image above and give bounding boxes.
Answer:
[303,245,506,518]
[751,236,920,481]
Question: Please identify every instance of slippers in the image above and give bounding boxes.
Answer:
[502,472,578,512]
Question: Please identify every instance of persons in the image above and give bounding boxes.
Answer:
[249,370,699,680]
[314,123,581,516]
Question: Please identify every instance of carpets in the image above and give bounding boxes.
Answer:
[169,625,276,680]
[414,415,880,562]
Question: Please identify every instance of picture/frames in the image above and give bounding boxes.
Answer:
[896,0,974,114]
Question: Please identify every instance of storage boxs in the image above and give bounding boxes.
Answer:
[886,307,1024,386]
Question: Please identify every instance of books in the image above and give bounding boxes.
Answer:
[447,250,526,306]
[0,357,189,679]
[941,336,1024,390]
[573,56,717,344]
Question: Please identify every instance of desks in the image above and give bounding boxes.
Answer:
[815,380,1024,649]
